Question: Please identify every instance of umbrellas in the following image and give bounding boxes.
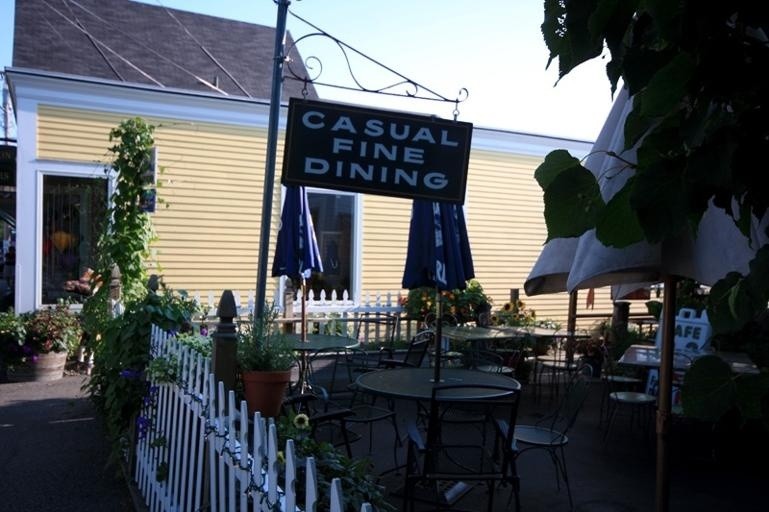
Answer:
[522,77,769,512]
[403,192,476,386]
[271,186,324,415]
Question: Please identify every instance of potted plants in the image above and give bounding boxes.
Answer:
[237,301,293,419]
[18,307,79,383]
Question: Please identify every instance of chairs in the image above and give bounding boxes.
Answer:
[276,321,768,471]
[512,363,594,510]
[401,383,523,510]
